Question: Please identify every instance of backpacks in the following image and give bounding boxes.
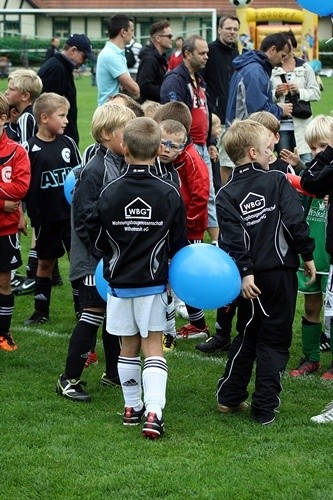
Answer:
[125,42,136,68]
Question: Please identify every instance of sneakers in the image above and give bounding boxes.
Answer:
[162,333,177,352]
[56,373,91,402]
[10,274,27,287]
[102,371,121,387]
[176,323,210,339]
[320,363,333,379]
[13,278,36,295]
[23,310,49,325]
[195,337,231,353]
[52,277,63,286]
[84,351,99,368]
[289,360,321,377]
[142,411,166,439]
[123,402,145,426]
[0,333,18,352]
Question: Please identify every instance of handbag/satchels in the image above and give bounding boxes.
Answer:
[279,74,312,119]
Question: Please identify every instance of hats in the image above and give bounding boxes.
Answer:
[66,33,97,61]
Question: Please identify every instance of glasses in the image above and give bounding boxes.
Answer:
[159,34,172,39]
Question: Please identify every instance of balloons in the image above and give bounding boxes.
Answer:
[295,0,333,16]
[169,243,241,310]
[64,164,81,204]
[95,257,110,301]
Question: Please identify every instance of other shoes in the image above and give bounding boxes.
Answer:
[217,403,248,412]
[175,305,189,319]
[319,338,331,351]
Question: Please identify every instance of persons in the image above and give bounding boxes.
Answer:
[0,15,332,435]
